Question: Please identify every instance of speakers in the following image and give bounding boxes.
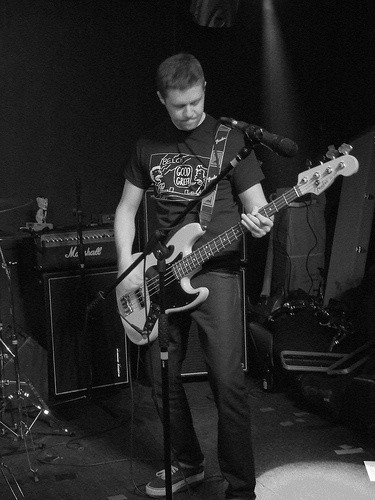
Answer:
[132,187,249,380]
[0,228,49,411]
[30,264,131,401]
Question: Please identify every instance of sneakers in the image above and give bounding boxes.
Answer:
[146,462,204,497]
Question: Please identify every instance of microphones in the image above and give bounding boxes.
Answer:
[219,117,299,161]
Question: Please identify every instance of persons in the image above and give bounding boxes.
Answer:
[114,54,274,500]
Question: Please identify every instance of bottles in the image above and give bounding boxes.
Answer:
[263,367,272,392]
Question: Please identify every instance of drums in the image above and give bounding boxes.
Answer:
[264,289,336,367]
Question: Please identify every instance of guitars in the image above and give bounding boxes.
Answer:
[114,143,358,345]
